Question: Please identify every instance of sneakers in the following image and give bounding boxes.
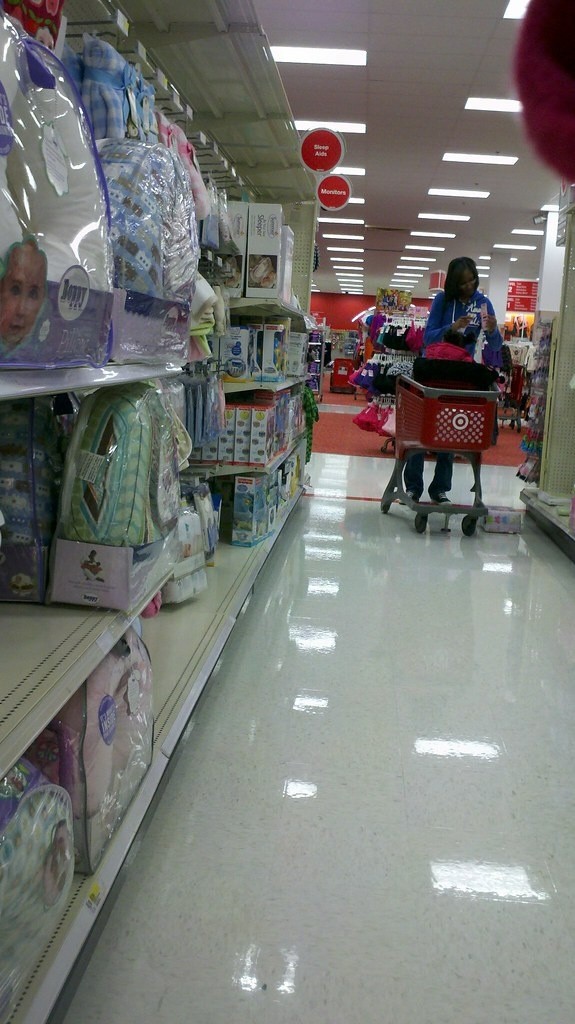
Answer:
[431,492,452,506]
[399,490,418,505]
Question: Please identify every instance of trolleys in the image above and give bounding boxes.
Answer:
[379,373,501,537]
[495,364,526,433]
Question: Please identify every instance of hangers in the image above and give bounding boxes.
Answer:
[369,353,418,363]
[390,316,428,328]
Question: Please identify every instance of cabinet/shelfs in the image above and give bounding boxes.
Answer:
[253,27,322,591]
[158,1,267,758]
[519,183,575,565]
[0,1,173,1024]
[501,312,536,433]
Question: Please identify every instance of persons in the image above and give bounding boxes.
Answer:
[0,241,46,345]
[399,257,503,504]
[518,317,527,337]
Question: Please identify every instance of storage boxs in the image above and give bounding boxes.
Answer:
[188,202,308,547]
